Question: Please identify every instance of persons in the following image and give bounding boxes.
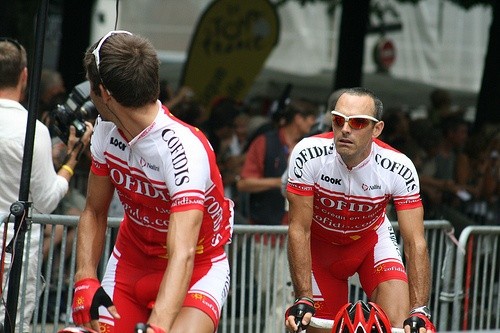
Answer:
[72,30,234,333]
[0,40,93,333]
[285,89,431,333]
[32,68,500,324]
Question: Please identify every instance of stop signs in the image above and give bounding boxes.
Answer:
[372,41,396,69]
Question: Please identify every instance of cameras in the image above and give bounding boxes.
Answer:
[51,79,97,145]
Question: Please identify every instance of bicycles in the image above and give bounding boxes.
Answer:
[58,300,155,333]
[291,303,426,333]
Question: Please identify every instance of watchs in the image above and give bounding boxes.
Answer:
[408,306,431,320]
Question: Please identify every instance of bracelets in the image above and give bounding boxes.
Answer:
[62,165,74,176]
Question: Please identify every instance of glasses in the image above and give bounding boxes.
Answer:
[93,28,134,96]
[330,110,380,130]
[0,37,22,66]
